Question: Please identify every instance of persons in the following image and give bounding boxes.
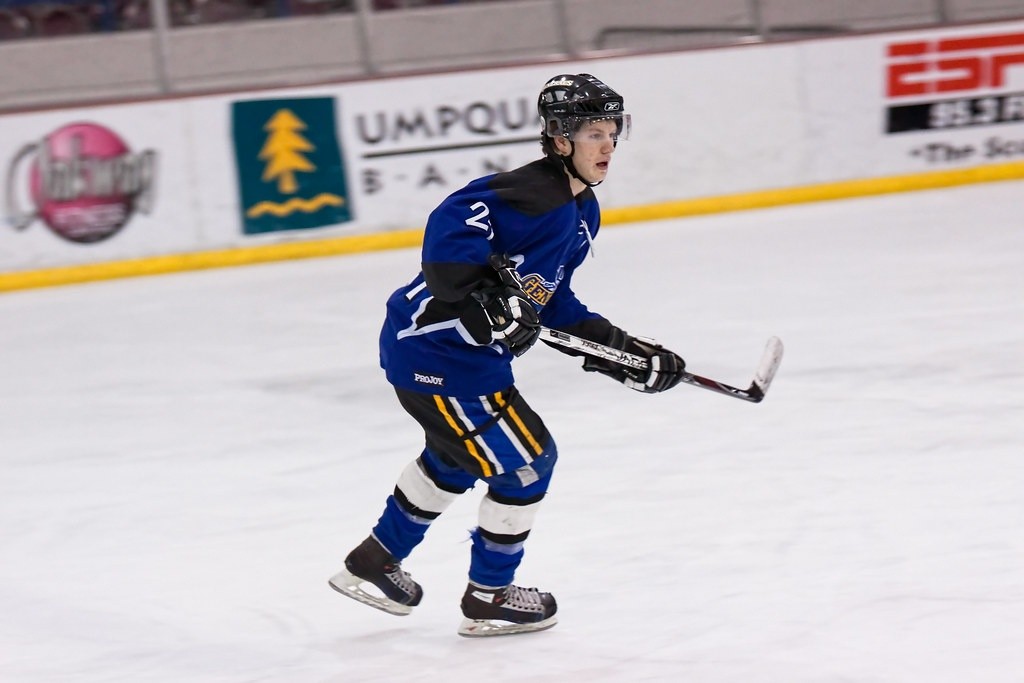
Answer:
[328,74,685,637]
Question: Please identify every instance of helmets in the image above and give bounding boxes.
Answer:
[537,72,632,141]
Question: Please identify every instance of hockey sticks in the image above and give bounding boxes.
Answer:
[496,313,787,405]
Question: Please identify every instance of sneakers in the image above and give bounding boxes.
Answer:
[327,534,423,615]
[456,581,559,635]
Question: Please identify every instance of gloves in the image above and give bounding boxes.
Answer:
[583,326,686,393]
[472,250,542,357]
